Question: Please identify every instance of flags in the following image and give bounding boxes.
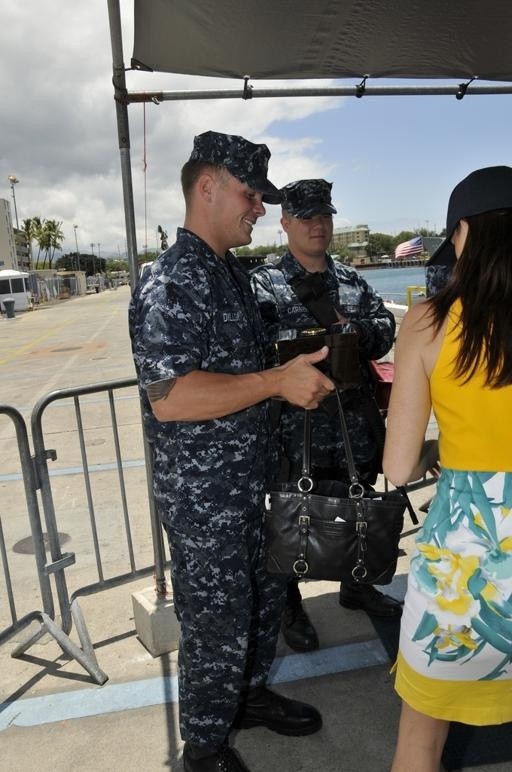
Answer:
[394,235,425,259]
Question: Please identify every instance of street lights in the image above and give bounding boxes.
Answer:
[8,175,24,272]
[73,223,81,271]
[91,242,102,275]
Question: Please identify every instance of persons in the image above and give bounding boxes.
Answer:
[381,165,511,771]
[251,176,404,651]
[126,129,337,771]
[425,264,453,299]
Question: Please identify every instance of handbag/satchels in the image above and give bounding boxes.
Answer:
[264,479,406,586]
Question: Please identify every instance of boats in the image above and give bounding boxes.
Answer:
[367,300,412,386]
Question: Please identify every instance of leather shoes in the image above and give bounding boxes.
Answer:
[179,743,244,770]
[341,589,397,612]
[280,601,320,651]
[233,691,321,735]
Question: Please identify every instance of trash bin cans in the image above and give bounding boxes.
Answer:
[3,298,15,318]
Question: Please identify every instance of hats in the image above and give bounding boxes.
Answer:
[191,130,286,204]
[280,179,338,218]
[425,165,510,265]
